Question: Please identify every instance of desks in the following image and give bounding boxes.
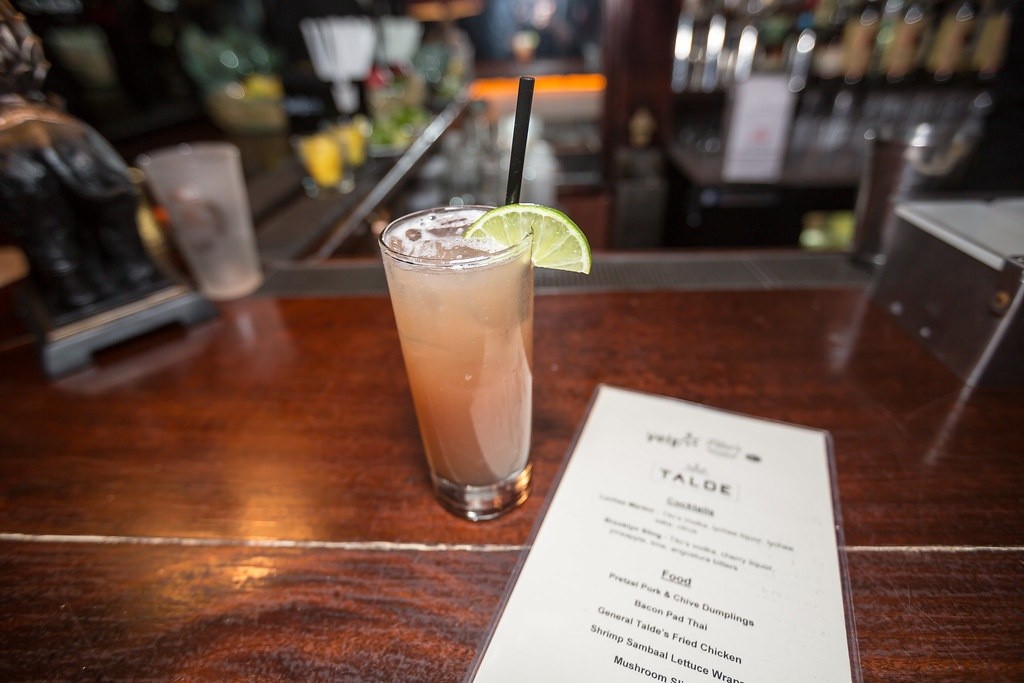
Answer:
[0,251,1024,683]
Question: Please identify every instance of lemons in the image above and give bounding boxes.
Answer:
[461,203,592,275]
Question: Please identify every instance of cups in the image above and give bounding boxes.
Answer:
[378,204,535,522]
[817,0,1015,80]
[137,141,264,301]
[849,122,944,269]
